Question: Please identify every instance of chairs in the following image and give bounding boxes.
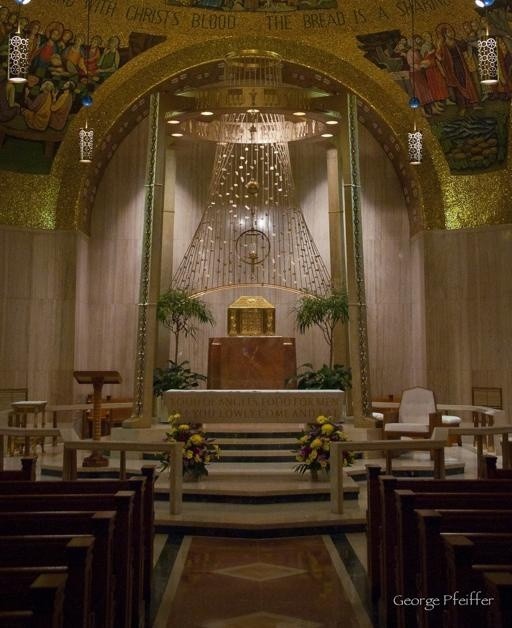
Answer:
[383,384,447,438]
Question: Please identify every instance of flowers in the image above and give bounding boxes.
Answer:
[158,413,219,476]
[291,415,356,479]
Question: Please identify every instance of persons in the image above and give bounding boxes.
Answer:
[1,6,122,134]
[380,2,512,118]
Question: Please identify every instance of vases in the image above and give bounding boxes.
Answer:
[183,469,201,481]
[312,457,330,480]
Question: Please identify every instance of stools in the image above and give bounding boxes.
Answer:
[441,415,463,447]
[7,399,49,455]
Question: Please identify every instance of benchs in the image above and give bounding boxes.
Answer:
[365,463,512,627]
[1,466,159,627]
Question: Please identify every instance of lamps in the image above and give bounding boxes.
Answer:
[76,0,97,167]
[403,2,427,165]
[471,0,505,86]
[6,0,31,82]
